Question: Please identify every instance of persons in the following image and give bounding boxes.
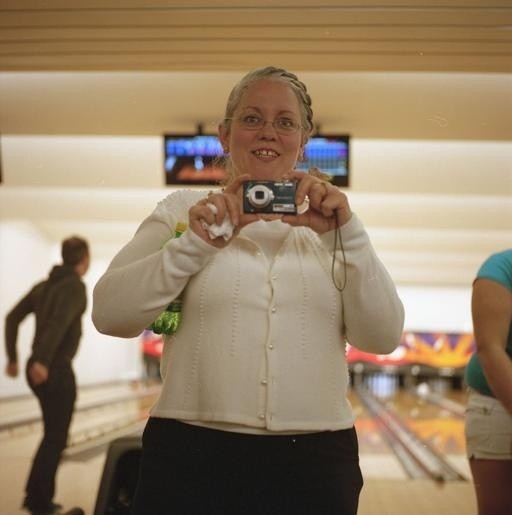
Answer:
[3,236,91,515]
[462,250,511,514]
[89,66,407,515]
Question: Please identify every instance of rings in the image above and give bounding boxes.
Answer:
[320,182,328,195]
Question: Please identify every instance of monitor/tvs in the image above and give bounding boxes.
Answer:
[165,134,232,185]
[295,135,349,186]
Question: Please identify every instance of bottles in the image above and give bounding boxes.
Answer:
[143,219,189,336]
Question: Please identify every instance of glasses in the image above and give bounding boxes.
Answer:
[223,115,304,135]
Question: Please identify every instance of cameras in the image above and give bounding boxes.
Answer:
[243,179,298,214]
